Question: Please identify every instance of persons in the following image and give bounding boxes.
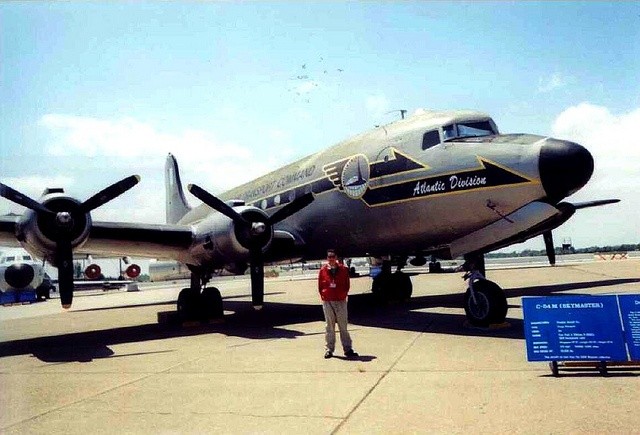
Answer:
[318,249,359,360]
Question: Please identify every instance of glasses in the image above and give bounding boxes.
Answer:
[328,255,335,257]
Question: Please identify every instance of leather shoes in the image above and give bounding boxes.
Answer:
[348,352,358,357]
[325,350,332,358]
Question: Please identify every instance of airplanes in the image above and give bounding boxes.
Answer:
[0,110,618,325]
[0,251,140,306]
